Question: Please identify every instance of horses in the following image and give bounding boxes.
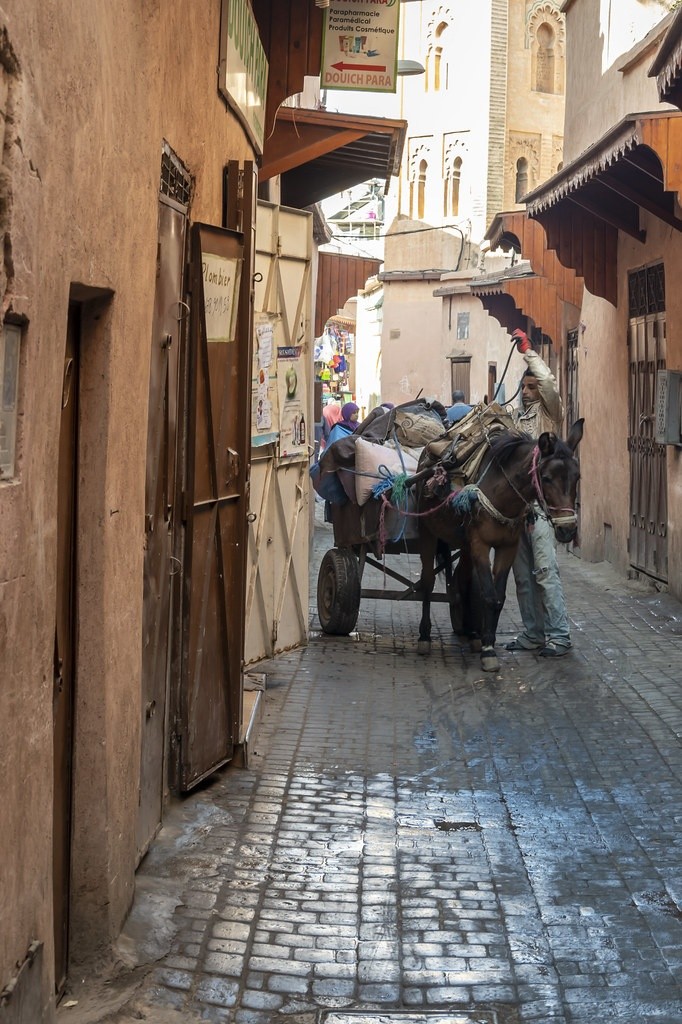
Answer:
[414,416,585,671]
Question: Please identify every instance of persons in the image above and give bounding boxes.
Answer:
[322,398,344,449]
[506,329,575,657]
[324,402,363,452]
[445,390,473,423]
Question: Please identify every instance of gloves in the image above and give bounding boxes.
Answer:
[512,329,531,353]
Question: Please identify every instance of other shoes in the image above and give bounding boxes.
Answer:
[539,642,574,657]
[505,641,524,650]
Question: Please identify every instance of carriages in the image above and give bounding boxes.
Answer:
[317,388,584,672]
